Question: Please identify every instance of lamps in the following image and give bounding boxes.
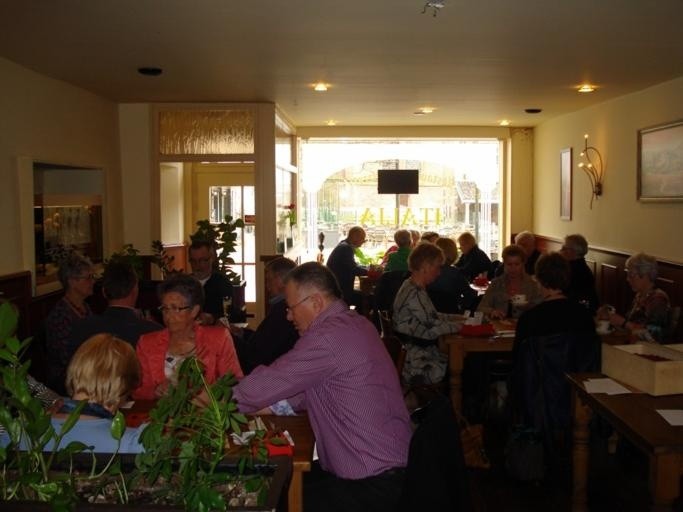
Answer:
[579,133,603,208]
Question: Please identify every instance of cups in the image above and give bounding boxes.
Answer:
[597,320,611,330]
[513,294,526,301]
[222,295,231,316]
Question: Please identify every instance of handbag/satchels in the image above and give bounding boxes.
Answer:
[457,416,493,470]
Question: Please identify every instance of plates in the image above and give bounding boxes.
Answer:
[598,328,617,337]
[510,300,528,306]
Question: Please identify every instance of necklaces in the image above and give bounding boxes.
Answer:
[65,296,90,316]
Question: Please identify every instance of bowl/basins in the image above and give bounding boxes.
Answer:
[368,271,382,278]
[460,324,494,336]
[475,277,488,285]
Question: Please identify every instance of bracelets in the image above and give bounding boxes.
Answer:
[622,319,628,328]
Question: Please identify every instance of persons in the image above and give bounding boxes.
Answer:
[73,261,164,347]
[188,241,239,324]
[2,334,146,453]
[425,238,472,313]
[561,235,595,302]
[505,251,603,417]
[383,230,415,272]
[386,230,419,252]
[130,273,244,399]
[598,252,673,343]
[328,227,372,314]
[421,232,439,244]
[496,231,543,277]
[455,232,492,281]
[44,255,95,376]
[393,241,485,427]
[230,263,416,511]
[477,245,543,319]
[231,257,300,371]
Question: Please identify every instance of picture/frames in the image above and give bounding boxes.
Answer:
[636,119,683,203]
[559,148,573,220]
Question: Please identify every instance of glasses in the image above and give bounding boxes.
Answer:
[71,273,96,281]
[626,272,639,279]
[188,257,210,264]
[285,295,311,315]
[157,305,190,314]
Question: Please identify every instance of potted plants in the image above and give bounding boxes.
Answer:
[0,300,293,511]
[197,216,247,308]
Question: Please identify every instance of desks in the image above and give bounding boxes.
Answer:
[122,407,316,511]
[432,320,682,511]
[359,275,377,321]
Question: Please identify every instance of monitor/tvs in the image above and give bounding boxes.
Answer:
[377,169,419,194]
